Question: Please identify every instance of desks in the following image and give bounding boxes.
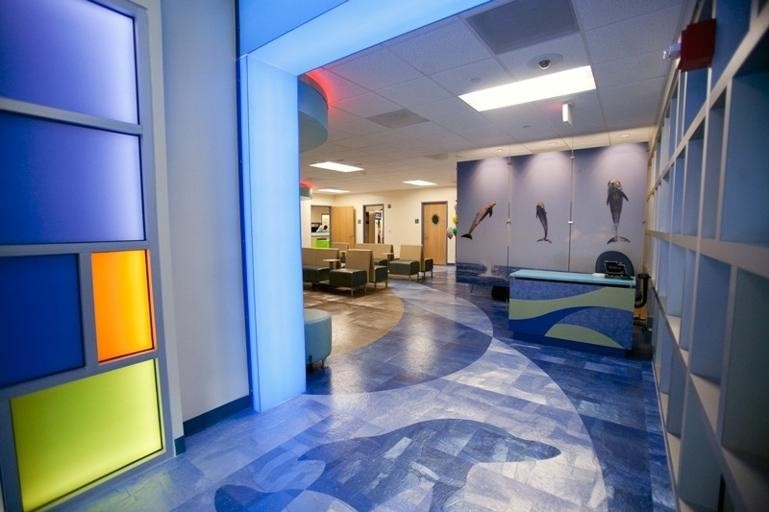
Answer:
[505,266,636,358]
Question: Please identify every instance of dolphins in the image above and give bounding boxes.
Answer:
[535,202,553,244]
[606,178,631,244]
[461,199,496,240]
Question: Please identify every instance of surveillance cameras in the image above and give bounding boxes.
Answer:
[538,59,552,70]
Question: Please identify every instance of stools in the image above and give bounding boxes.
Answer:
[302,308,333,369]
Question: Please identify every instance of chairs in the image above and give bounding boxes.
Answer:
[301,242,434,299]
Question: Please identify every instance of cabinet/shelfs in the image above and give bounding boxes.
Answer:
[639,0,768,512]
